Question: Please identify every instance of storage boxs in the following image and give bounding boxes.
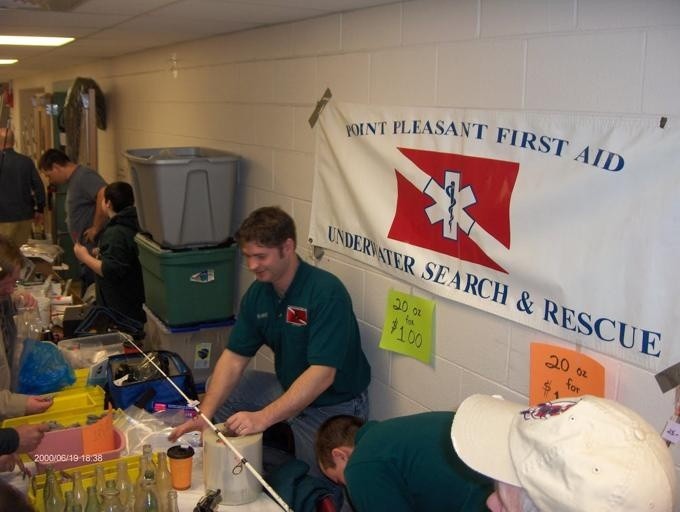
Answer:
[1,332,133,478]
[121,147,240,404]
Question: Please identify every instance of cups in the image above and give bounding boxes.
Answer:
[167,445,194,489]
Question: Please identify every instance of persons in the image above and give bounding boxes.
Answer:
[0,234,54,512]
[167,206,371,512]
[37,148,110,305]
[0,127,46,246]
[315,410,496,512]
[73,181,147,341]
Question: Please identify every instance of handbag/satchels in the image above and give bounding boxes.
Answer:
[107,349,199,414]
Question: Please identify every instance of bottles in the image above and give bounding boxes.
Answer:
[44,445,179,512]
